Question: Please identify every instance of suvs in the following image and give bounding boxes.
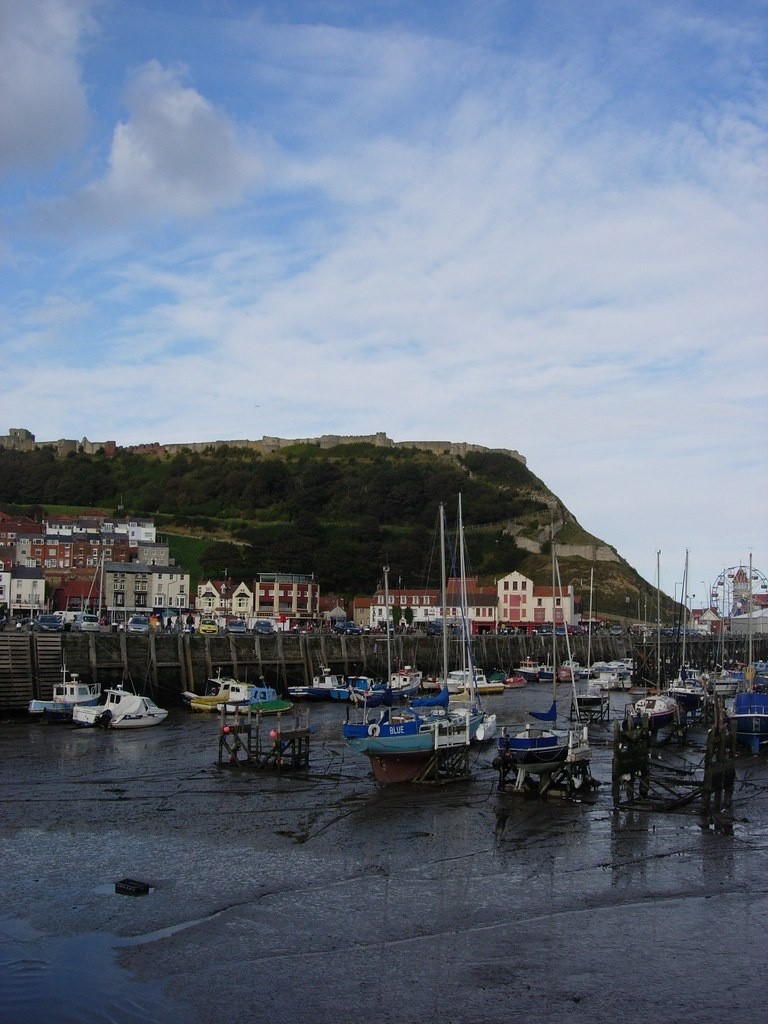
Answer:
[334,621,362,636]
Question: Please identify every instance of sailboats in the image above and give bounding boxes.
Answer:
[26,487,767,787]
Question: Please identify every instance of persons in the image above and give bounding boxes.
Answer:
[498,621,518,635]
[166,614,195,632]
[99,615,111,626]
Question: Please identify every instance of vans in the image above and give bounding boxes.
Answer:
[70,613,103,633]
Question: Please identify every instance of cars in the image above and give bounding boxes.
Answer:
[224,618,247,633]
[32,614,64,633]
[538,623,553,634]
[199,618,218,635]
[555,626,568,636]
[252,620,275,634]
[126,616,149,636]
[0,616,7,631]
[610,625,622,636]
